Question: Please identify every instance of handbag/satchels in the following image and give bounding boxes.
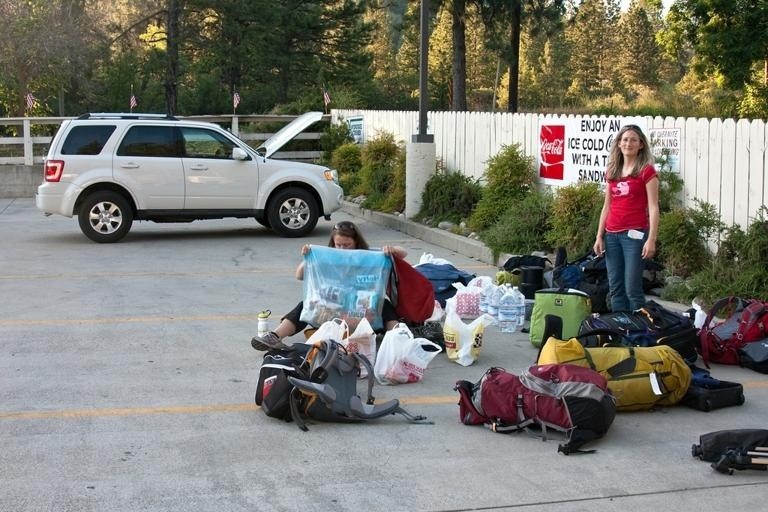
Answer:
[414,264,475,308]
[692,429,768,475]
[738,339,766,375]
[529,288,593,348]
[501,251,609,320]
[536,336,692,413]
[442,299,494,367]
[387,257,435,325]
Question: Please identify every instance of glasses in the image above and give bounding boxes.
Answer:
[331,222,359,233]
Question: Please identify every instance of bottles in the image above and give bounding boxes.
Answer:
[480,283,525,332]
[257,310,271,337]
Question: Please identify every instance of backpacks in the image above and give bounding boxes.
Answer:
[699,296,766,368]
[578,300,700,363]
[455,363,617,457]
[254,338,434,433]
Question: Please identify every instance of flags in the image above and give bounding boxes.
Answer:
[130,90,138,109]
[26,89,34,110]
[233,88,241,109]
[323,83,331,107]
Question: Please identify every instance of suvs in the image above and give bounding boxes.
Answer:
[33,110,346,242]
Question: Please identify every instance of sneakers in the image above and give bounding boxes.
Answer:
[250,332,283,351]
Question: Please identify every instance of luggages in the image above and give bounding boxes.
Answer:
[657,333,745,413]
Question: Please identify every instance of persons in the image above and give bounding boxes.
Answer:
[251,220,408,350]
[593,124,659,312]
